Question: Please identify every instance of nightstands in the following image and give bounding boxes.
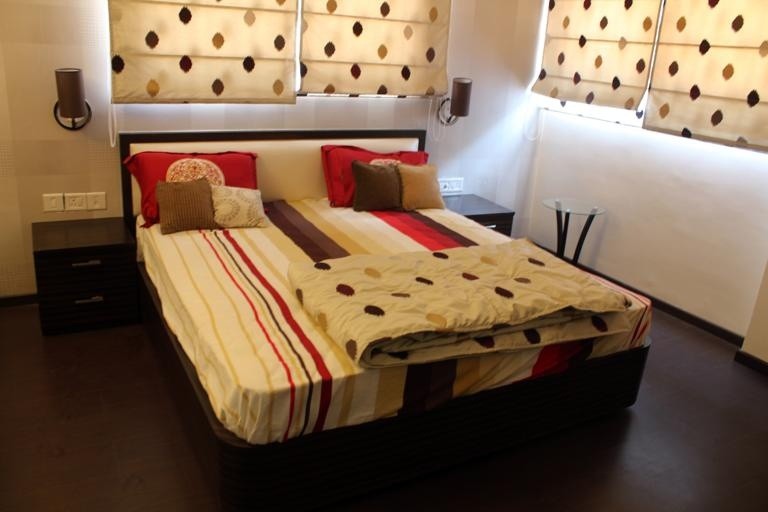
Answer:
[442,194,516,237]
[32,217,137,338]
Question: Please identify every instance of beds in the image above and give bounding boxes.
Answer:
[119,129,652,512]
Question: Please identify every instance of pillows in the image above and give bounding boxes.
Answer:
[398,164,445,212]
[122,151,270,229]
[156,177,216,234]
[209,183,270,228]
[351,160,401,212]
[321,144,429,208]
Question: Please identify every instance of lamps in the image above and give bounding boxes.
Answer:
[438,77,473,127]
[53,69,92,131]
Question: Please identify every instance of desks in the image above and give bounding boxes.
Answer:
[542,197,607,265]
